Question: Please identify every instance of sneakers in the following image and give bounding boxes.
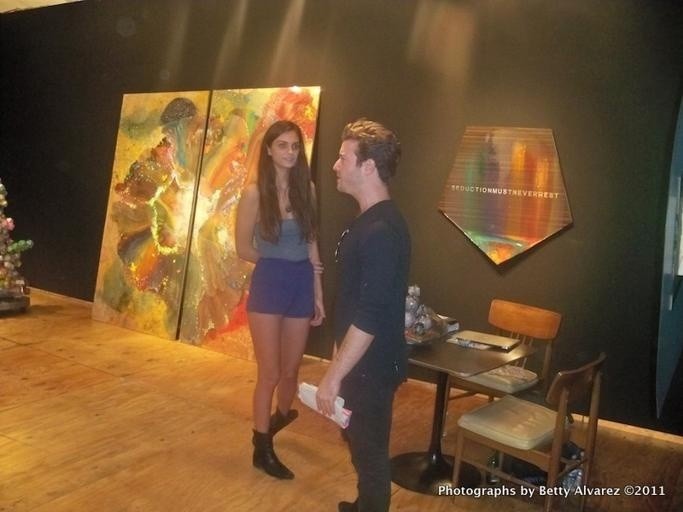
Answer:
[338,499,361,512]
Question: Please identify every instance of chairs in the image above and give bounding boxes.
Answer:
[438,295,564,440]
[446,348,610,511]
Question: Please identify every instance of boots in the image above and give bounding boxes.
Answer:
[251,427,295,480]
[269,406,298,434]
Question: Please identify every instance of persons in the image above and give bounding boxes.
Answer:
[314,117,415,512]
[234,118,326,480]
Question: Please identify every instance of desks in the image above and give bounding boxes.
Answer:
[386,333,541,498]
